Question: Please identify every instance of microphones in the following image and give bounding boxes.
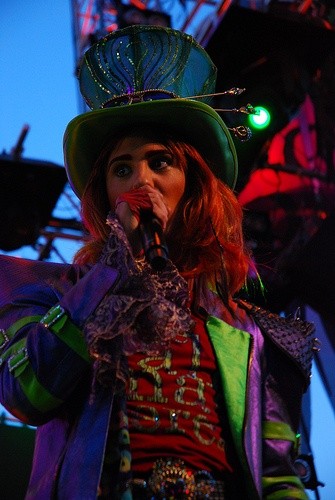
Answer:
[139,209,170,269]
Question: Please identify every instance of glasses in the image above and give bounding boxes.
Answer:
[98,88,181,109]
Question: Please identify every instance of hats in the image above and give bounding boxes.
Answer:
[63,26,255,201]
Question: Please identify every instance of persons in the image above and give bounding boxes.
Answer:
[0,24,322,500]
